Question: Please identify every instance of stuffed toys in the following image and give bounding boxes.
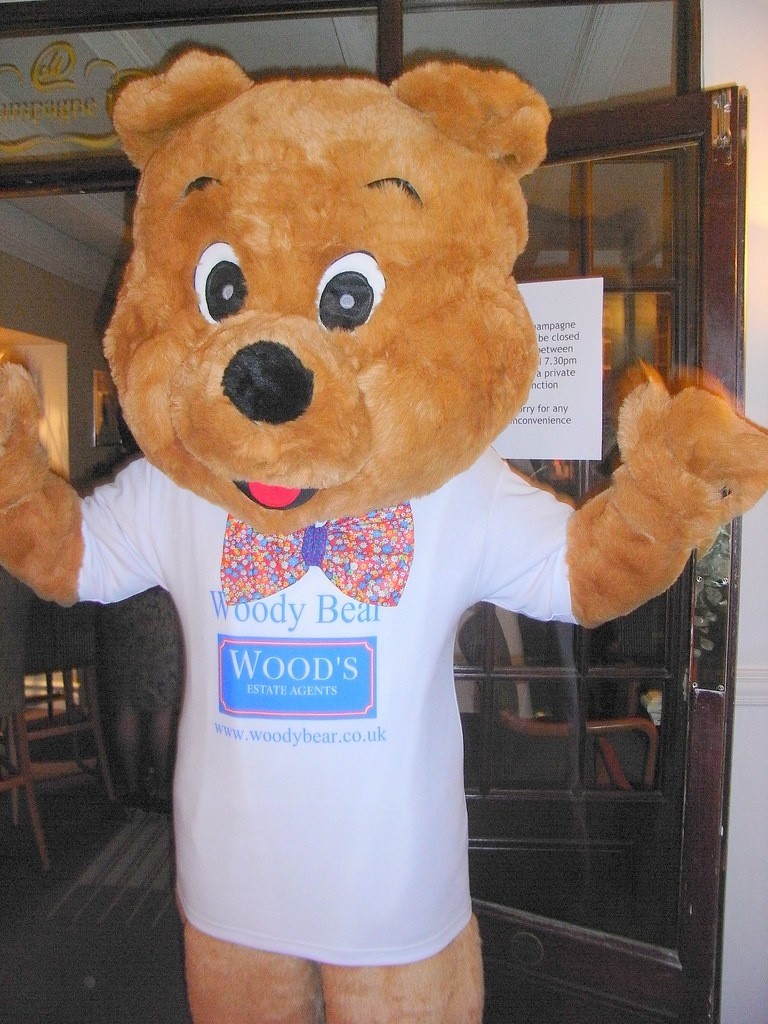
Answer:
[0,50,768,1024]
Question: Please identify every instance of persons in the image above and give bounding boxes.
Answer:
[102,585,181,796]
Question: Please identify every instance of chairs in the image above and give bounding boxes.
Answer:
[466,606,660,789]
[0,572,117,881]
